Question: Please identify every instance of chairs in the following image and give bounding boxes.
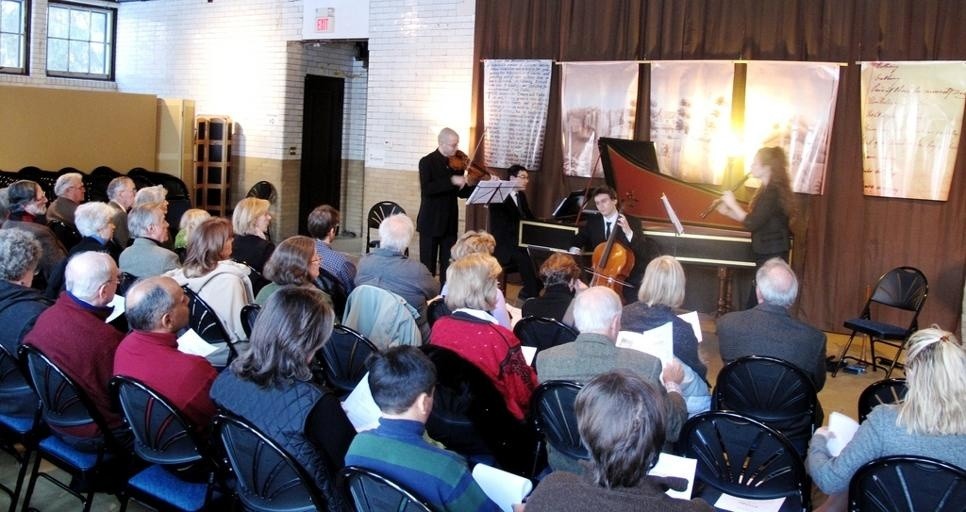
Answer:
[230,252,271,300]
[105,373,221,512]
[712,357,821,498]
[676,410,808,512]
[207,410,338,512]
[16,345,139,512]
[107,270,144,336]
[830,263,932,385]
[173,280,241,375]
[239,304,335,390]
[336,464,442,511]
[365,200,412,260]
[30,269,48,287]
[0,344,105,511]
[487,207,543,302]
[414,343,515,431]
[318,323,389,395]
[512,316,582,375]
[313,265,347,320]
[229,179,275,243]
[859,379,910,425]
[847,454,966,511]
[2,161,193,262]
[624,238,660,305]
[537,381,659,472]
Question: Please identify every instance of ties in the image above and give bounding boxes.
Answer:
[604,221,614,244]
[514,193,524,219]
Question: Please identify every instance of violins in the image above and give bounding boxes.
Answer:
[449,151,509,187]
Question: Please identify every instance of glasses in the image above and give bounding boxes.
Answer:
[36,192,45,199]
[107,276,125,283]
[511,175,531,178]
[120,189,138,193]
[311,255,323,262]
[27,268,40,274]
[444,142,459,147]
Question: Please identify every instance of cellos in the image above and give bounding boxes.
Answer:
[592,190,634,304]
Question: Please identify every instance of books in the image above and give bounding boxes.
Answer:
[676,311,702,342]
[506,302,521,332]
[713,493,786,512]
[520,346,537,367]
[615,321,673,387]
[176,328,219,357]
[825,411,861,457]
[105,293,125,323]
[472,462,532,511]
[646,452,697,501]
[466,180,517,205]
[340,370,383,433]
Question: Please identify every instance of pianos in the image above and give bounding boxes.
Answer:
[518,137,799,313]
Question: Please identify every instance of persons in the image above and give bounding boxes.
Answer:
[113,275,220,482]
[354,212,440,345]
[175,208,212,268]
[806,323,966,496]
[119,202,182,278]
[45,201,120,298]
[161,215,256,344]
[345,343,527,512]
[441,230,511,330]
[126,185,173,249]
[230,197,276,299]
[46,173,86,224]
[22,251,131,453]
[488,164,545,301]
[253,235,340,326]
[521,252,588,329]
[0,186,11,229]
[107,176,137,249]
[430,252,541,420]
[308,205,357,294]
[209,287,359,511]
[619,254,712,388]
[521,366,717,512]
[0,180,70,287]
[713,146,795,310]
[567,185,647,305]
[0,227,57,418]
[415,127,468,295]
[536,286,687,476]
[710,257,828,459]
